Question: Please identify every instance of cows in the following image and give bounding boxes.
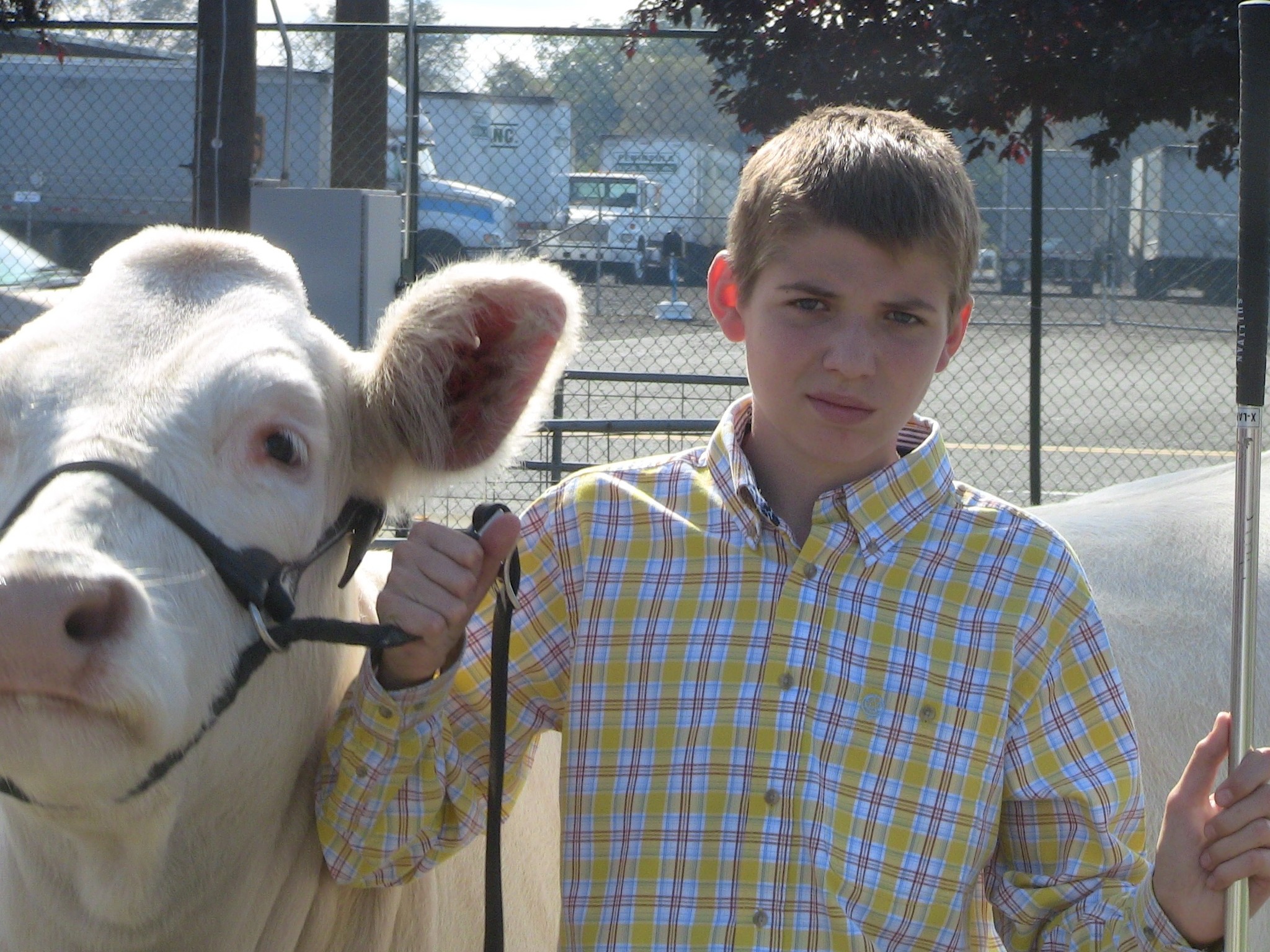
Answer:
[1,224,1270,952]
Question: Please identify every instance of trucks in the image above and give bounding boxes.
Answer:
[1130,142,1241,299]
[1,53,533,281]
[998,149,1113,297]
[538,132,743,278]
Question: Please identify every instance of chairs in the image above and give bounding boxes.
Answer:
[614,192,637,207]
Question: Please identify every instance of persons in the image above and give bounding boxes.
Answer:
[315,106,1270,952]
